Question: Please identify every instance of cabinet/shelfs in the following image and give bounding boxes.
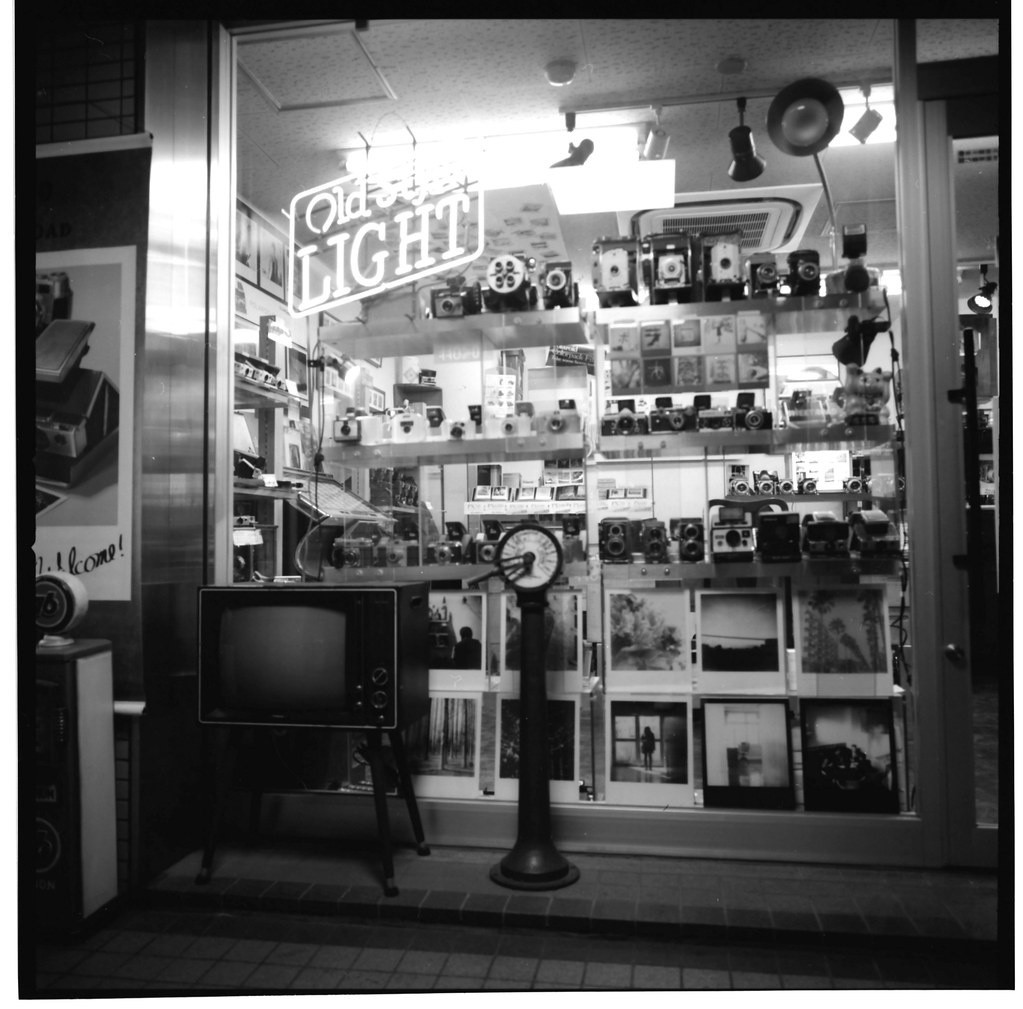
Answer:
[233,378,289,581]
[319,298,906,816]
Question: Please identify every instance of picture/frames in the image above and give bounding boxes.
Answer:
[428,593,486,692]
[494,695,579,800]
[602,694,695,810]
[701,702,798,809]
[791,579,894,696]
[602,588,696,696]
[412,694,482,796]
[316,359,386,414]
[802,699,900,814]
[694,589,788,697]
[502,594,584,694]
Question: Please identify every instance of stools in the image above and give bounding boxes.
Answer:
[197,700,437,898]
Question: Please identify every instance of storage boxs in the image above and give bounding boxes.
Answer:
[608,324,640,351]
[483,374,517,418]
[611,359,642,396]
[674,319,700,350]
[673,355,704,385]
[475,463,502,488]
[504,472,522,487]
[705,353,737,385]
[737,351,769,389]
[700,314,737,351]
[641,321,673,357]
[643,359,671,387]
[736,314,767,346]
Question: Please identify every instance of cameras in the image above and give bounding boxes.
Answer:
[333,221,902,568]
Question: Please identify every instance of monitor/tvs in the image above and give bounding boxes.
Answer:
[196,580,426,731]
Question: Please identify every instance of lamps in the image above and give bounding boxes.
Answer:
[831,313,889,368]
[643,104,670,158]
[967,265,998,315]
[848,86,881,142]
[728,99,765,182]
